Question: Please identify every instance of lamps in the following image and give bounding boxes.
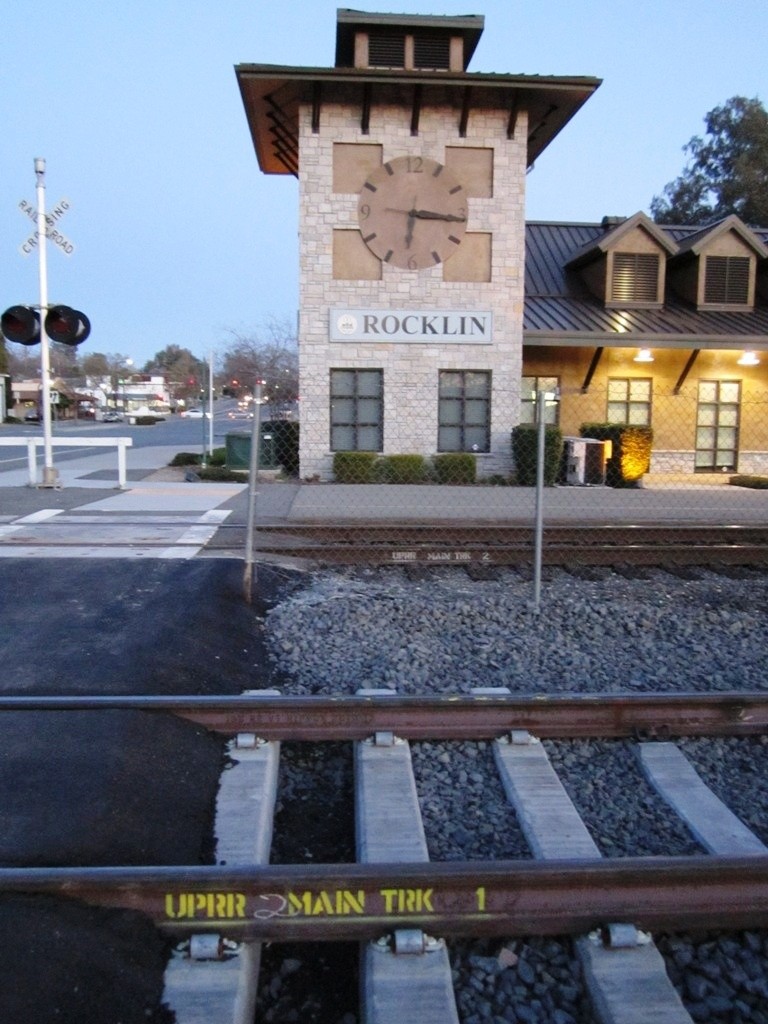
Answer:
[633,348,654,362]
[737,350,759,366]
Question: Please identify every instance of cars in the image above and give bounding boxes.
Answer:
[268,406,292,417]
[228,409,253,419]
[181,408,213,420]
[104,411,123,423]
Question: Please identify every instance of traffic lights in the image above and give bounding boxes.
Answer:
[61,310,91,345]
[45,304,78,341]
[20,311,41,346]
[1,303,36,342]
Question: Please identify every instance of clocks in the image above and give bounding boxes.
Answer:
[357,155,468,270]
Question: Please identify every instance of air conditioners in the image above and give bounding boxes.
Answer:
[558,436,610,487]
